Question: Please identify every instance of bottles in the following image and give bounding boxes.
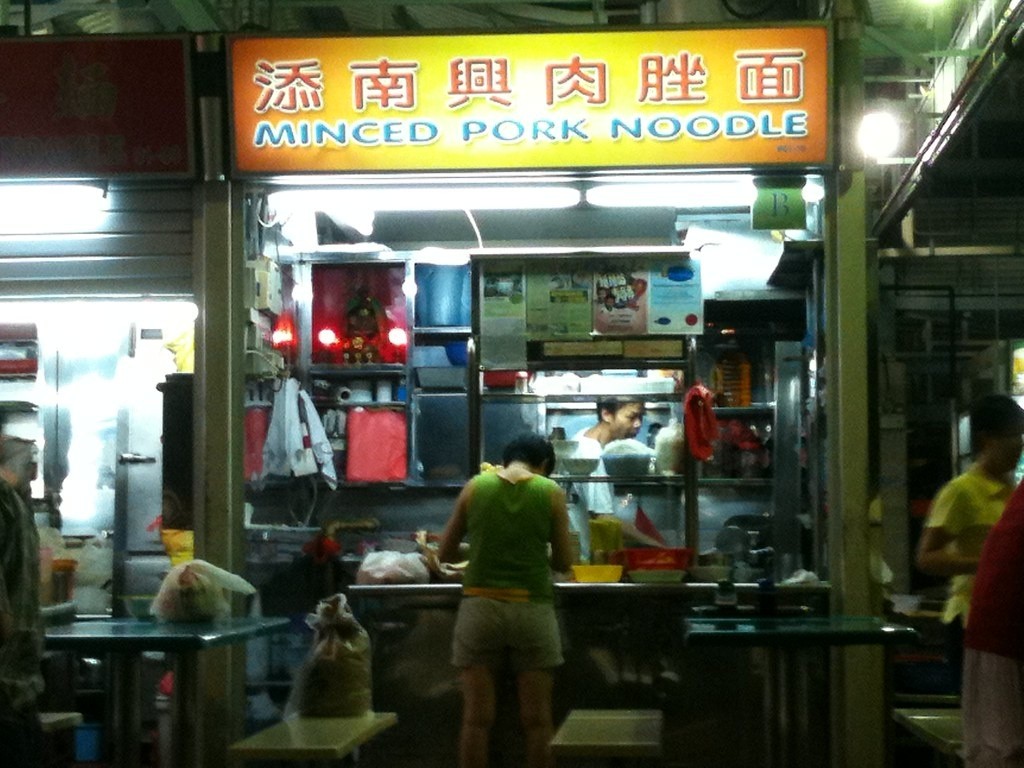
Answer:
[549,428,565,441]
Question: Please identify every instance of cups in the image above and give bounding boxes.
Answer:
[550,441,579,474]
[512,372,529,396]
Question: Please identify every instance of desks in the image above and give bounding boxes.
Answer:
[683,617,920,768]
[45,616,293,768]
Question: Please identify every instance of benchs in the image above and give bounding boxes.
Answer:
[228,709,399,768]
[40,713,83,731]
[891,707,962,768]
[551,710,666,768]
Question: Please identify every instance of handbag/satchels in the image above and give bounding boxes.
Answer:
[149,559,260,625]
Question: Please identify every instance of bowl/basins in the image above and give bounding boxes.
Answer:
[414,367,467,388]
[622,547,694,569]
[571,565,623,584]
[118,594,158,622]
[602,454,649,475]
[732,567,763,583]
[562,458,598,475]
[687,566,729,580]
[481,369,533,389]
[627,570,687,583]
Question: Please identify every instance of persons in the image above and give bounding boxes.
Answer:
[914,395,1024,768]
[437,431,571,768]
[0,477,47,768]
[561,396,666,564]
[600,294,618,313]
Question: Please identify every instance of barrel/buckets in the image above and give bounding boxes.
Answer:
[71,720,105,763]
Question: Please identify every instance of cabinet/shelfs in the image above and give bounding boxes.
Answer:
[699,405,777,487]
[466,336,699,582]
[294,252,417,487]
